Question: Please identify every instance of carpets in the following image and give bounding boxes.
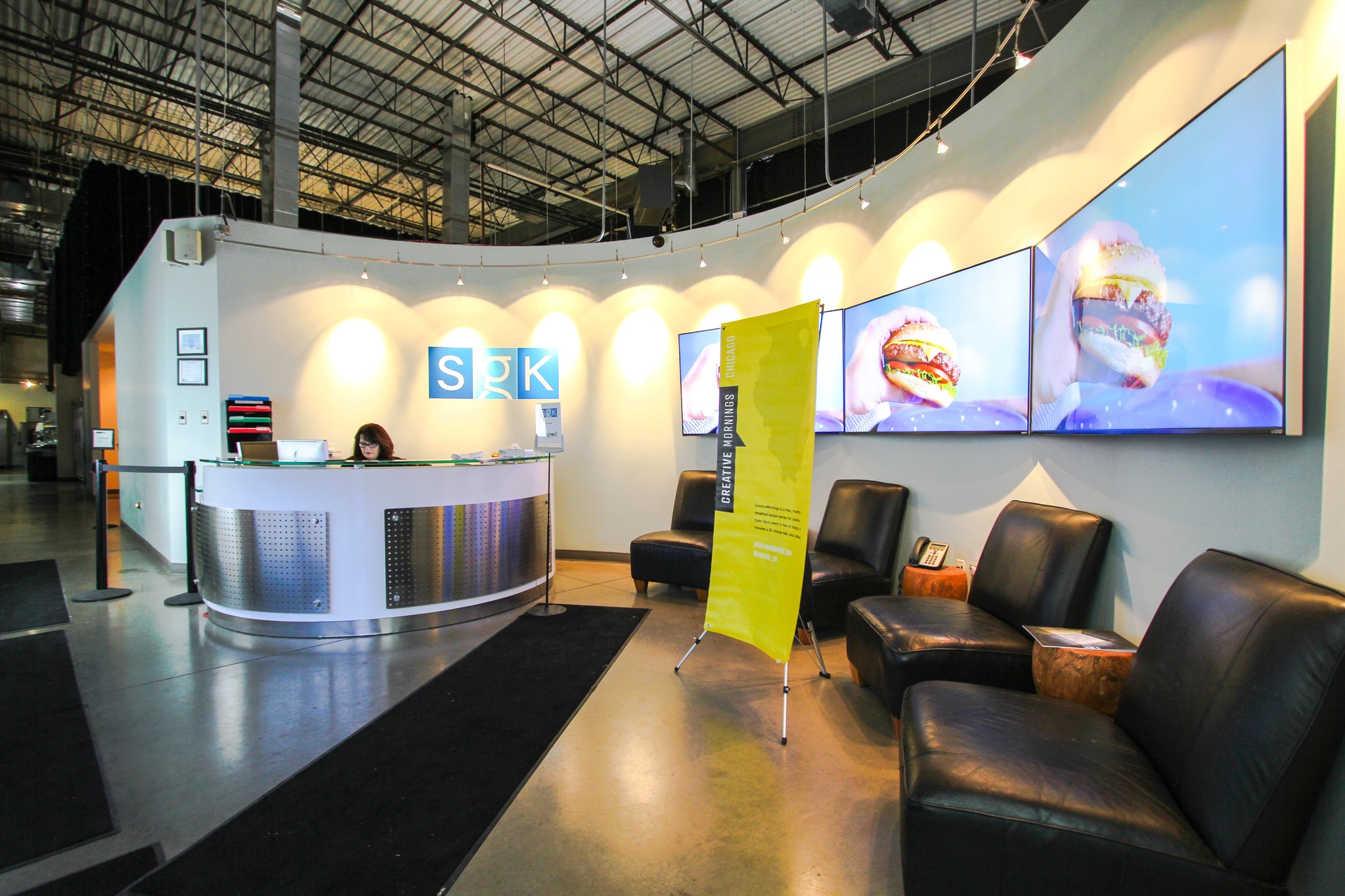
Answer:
[0,560,654,896]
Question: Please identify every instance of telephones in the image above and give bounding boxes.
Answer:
[907,536,949,570]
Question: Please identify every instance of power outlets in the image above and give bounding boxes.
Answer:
[957,558,966,571]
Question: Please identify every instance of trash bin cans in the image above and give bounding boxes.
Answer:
[25,444,58,482]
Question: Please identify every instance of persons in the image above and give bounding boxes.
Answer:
[1030,220,1143,411]
[340,423,410,467]
[681,342,721,425]
[845,305,942,418]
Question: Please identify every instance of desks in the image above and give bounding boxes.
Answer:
[903,564,968,603]
[1032,640,1136,715]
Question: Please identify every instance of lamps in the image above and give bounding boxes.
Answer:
[935,120,950,154]
[780,221,790,244]
[858,183,870,210]
[622,261,628,280]
[700,246,706,267]
[361,257,368,279]
[457,265,464,285]
[1012,23,1031,70]
[543,266,548,285]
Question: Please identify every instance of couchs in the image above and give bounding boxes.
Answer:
[631,470,717,601]
[844,500,1112,741]
[797,480,909,643]
[898,548,1345,896]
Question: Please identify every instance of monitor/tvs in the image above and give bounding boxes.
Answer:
[276,440,330,462]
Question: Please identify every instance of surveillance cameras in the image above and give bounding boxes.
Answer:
[652,235,665,248]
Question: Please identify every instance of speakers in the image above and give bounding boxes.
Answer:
[162,228,202,268]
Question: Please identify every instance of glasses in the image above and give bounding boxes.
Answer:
[358,442,379,448]
